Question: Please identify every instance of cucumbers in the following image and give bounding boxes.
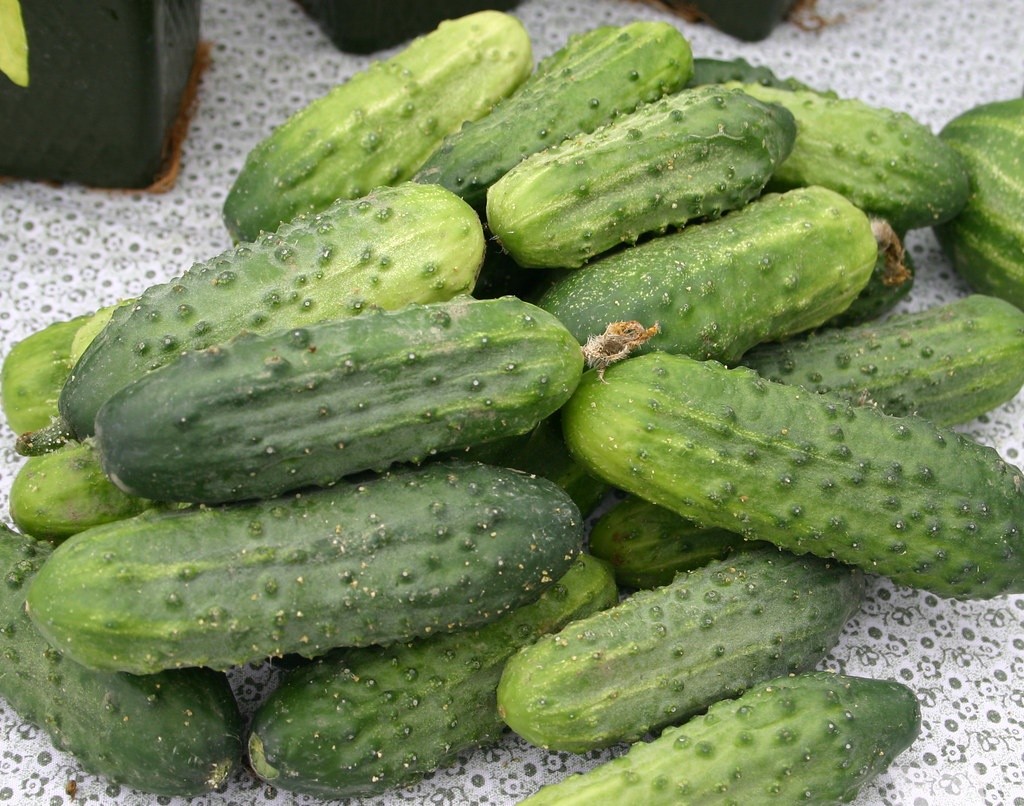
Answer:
[1,10,1024,806]
